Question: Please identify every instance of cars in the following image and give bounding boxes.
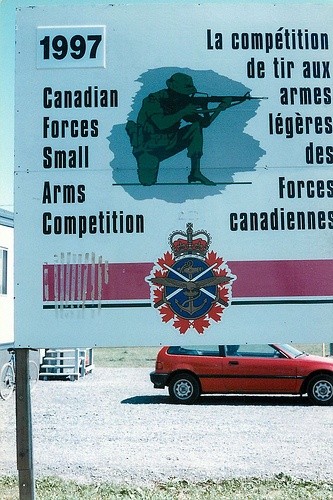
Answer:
[150,344,333,406]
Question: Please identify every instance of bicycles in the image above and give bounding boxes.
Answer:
[0,348,39,401]
